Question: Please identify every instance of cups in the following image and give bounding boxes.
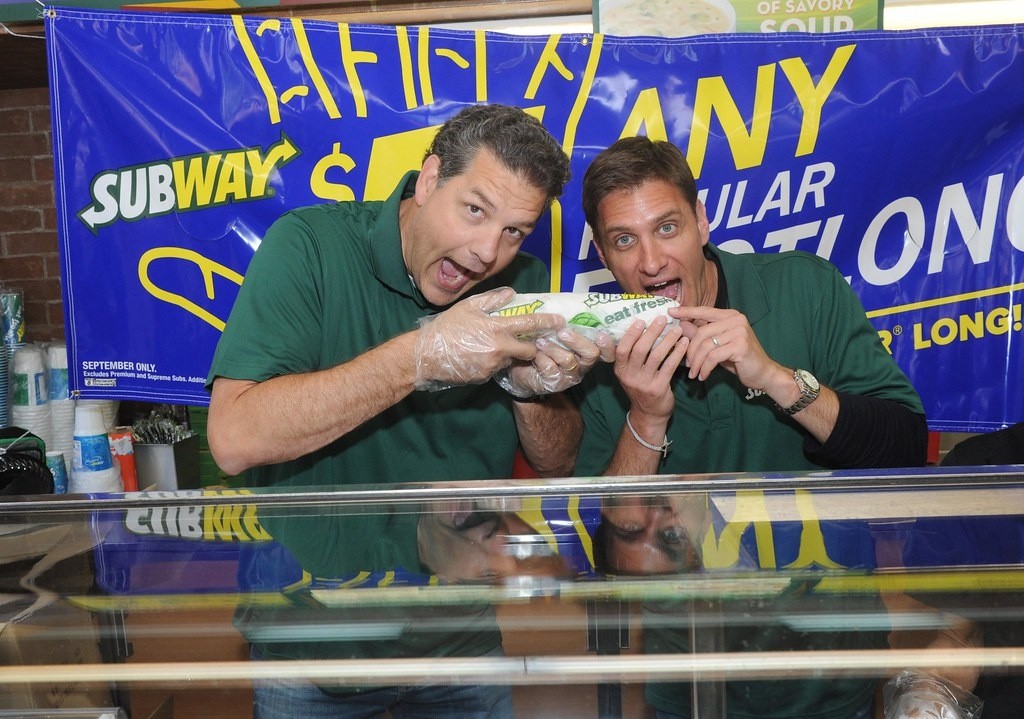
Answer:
[0,293,121,493]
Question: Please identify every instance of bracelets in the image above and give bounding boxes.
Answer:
[626,410,674,458]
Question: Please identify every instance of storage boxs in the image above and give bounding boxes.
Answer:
[132,433,201,492]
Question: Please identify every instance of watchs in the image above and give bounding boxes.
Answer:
[775,368,820,415]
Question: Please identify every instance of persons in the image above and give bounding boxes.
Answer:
[204,103,617,719]
[593,491,705,577]
[253,500,572,587]
[567,136,929,719]
[885,421,1024,719]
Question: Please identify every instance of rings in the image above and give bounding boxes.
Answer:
[566,362,578,371]
[711,336,719,347]
